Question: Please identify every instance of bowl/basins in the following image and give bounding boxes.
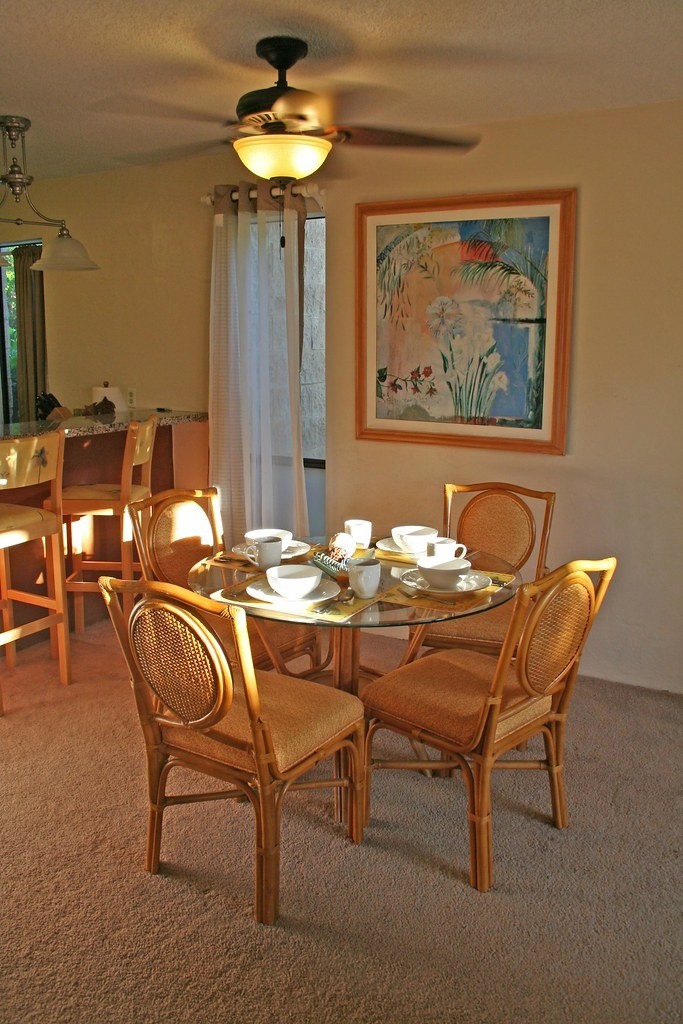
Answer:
[391,526,438,551]
[244,528,293,553]
[417,556,471,587]
[266,564,322,598]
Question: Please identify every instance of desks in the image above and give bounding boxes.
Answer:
[186,536,524,821]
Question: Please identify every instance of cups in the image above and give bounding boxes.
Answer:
[244,537,282,570]
[426,537,467,558]
[347,558,381,598]
[345,520,372,549]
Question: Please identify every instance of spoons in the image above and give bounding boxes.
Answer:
[318,589,355,613]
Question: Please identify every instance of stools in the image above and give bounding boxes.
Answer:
[42,415,159,657]
[0,429,73,714]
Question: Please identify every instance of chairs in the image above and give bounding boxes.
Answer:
[362,555,617,891]
[126,487,321,676]
[97,574,366,924]
[409,480,555,777]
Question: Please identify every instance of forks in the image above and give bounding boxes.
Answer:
[397,588,454,604]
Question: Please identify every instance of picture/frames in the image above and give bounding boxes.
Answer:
[354,187,580,454]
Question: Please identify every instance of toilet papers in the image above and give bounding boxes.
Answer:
[92,385,130,414]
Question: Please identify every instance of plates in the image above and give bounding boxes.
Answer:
[399,568,492,594]
[246,578,341,603]
[232,541,311,559]
[376,537,456,555]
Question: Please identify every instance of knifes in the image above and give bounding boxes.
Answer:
[34,390,61,419]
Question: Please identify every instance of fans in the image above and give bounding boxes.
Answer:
[86,34,483,170]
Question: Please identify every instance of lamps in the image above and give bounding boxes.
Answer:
[232,131,332,186]
[0,114,98,273]
